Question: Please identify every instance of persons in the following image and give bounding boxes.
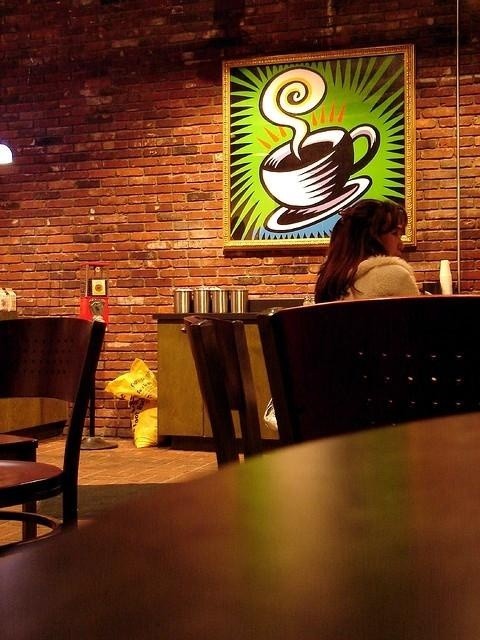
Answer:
[264,200,420,433]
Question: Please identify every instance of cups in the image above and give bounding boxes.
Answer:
[173,287,249,314]
[422,282,441,294]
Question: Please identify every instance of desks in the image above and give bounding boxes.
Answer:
[0,410,478,638]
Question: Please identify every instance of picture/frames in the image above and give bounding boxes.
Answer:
[221,40,419,255]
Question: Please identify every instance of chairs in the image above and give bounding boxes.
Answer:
[256,295,479,449]
[182,311,283,470]
[0,311,103,552]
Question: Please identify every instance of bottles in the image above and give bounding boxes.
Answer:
[302,295,315,306]
